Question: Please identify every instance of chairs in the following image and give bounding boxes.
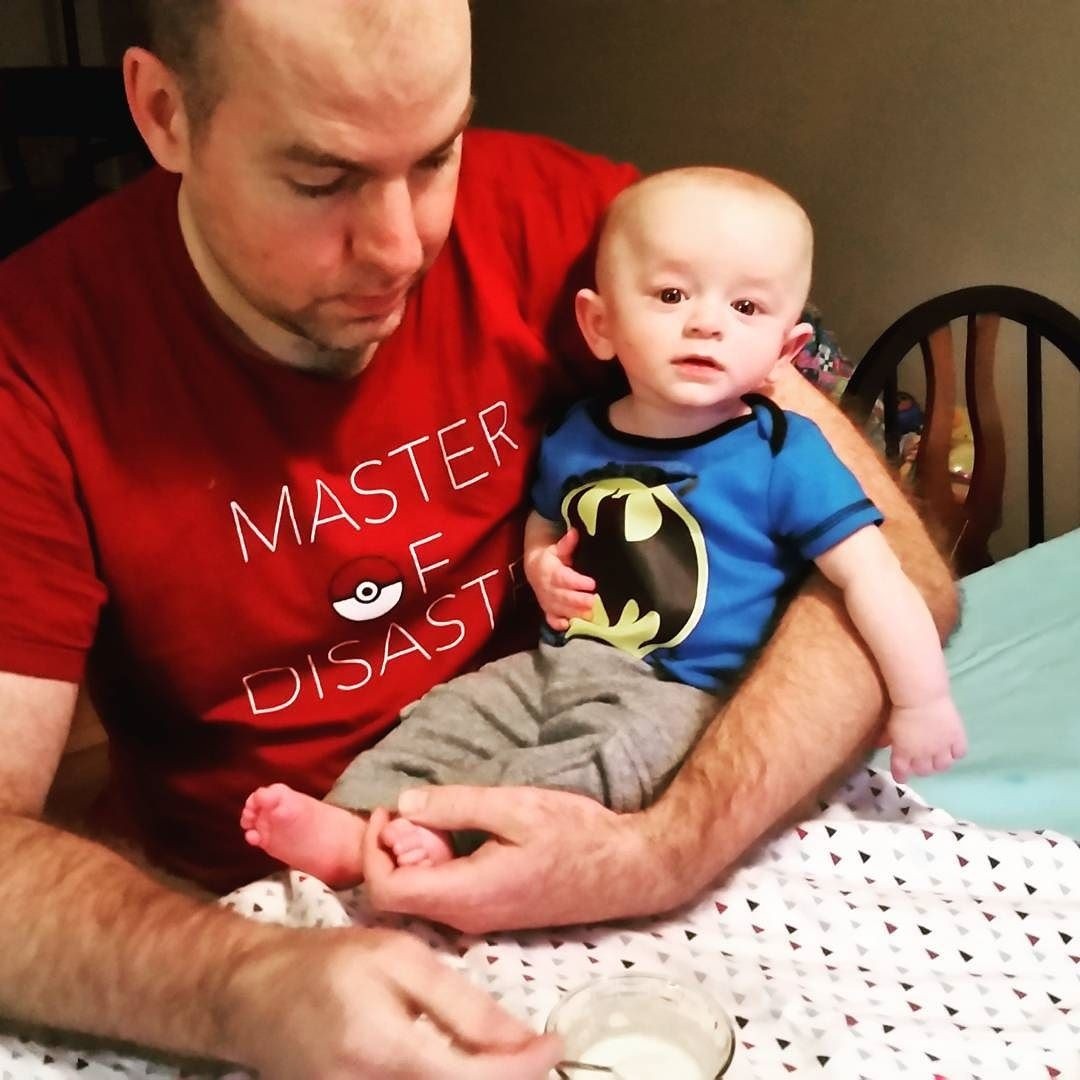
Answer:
[837,284,1080,581]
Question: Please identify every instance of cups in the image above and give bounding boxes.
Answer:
[547,975,737,1079]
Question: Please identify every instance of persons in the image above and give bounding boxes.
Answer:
[0,0,962,1080]
[242,166,972,878]
